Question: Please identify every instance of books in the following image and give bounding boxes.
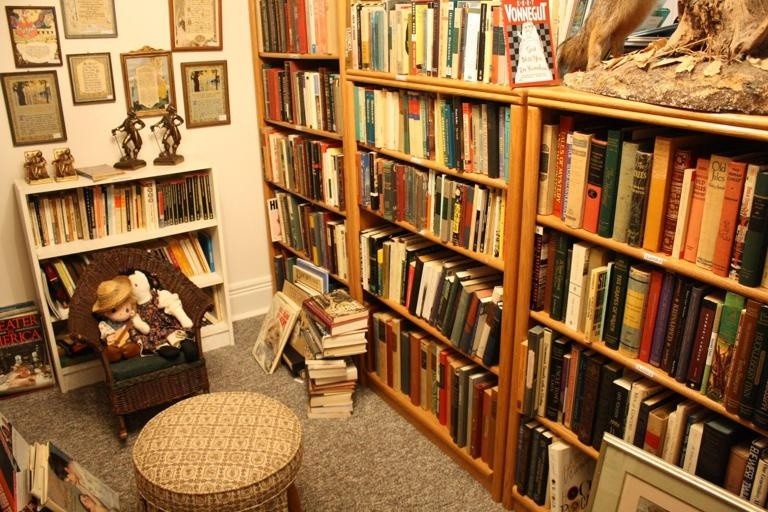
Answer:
[0,412,122,512]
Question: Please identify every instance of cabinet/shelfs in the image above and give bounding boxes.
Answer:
[250,1,351,307]
[339,5,501,500]
[12,159,233,392]
[500,4,767,510]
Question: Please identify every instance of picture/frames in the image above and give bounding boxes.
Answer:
[0,70,68,148]
[168,1,224,53]
[3,6,63,70]
[65,53,116,105]
[121,46,177,118]
[576,428,759,509]
[181,60,231,129]
[59,1,118,39]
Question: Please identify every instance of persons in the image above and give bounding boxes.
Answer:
[150,104,184,158]
[111,108,145,161]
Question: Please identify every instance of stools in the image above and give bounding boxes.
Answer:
[127,391,306,510]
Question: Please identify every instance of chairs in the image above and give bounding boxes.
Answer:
[65,247,215,442]
